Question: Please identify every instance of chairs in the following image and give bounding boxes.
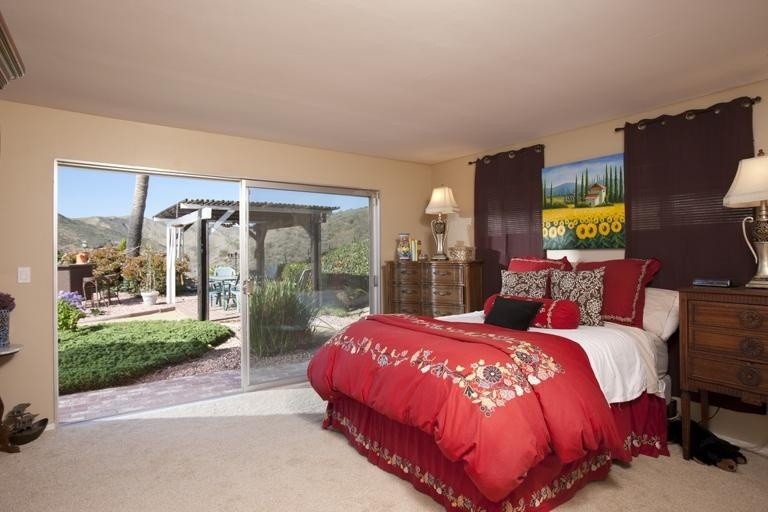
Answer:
[205,263,311,314]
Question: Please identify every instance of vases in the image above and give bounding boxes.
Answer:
[138,289,160,306]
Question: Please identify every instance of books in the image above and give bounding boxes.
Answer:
[687,279,744,288]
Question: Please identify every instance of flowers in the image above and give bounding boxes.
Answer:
[542,204,624,250]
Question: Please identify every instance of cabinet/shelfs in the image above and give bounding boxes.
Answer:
[677,282,768,463]
[385,259,490,319]
[81,273,112,311]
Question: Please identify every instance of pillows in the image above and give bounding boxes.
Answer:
[481,255,680,342]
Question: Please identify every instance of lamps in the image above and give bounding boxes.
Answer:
[423,184,460,261]
[719,147,767,289]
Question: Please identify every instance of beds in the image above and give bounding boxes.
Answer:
[305,255,680,512]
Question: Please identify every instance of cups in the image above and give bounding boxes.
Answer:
[410,239,421,262]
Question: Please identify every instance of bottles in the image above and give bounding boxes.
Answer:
[419,252,430,261]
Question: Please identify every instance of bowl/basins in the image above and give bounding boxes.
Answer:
[447,247,476,261]
